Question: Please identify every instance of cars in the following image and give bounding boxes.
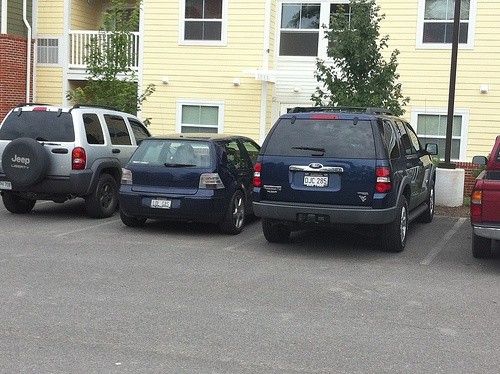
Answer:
[117,132,261,235]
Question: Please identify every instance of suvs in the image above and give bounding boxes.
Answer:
[0,102,153,218]
[251,106,439,252]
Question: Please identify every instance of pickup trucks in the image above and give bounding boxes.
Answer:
[469,135,500,258]
